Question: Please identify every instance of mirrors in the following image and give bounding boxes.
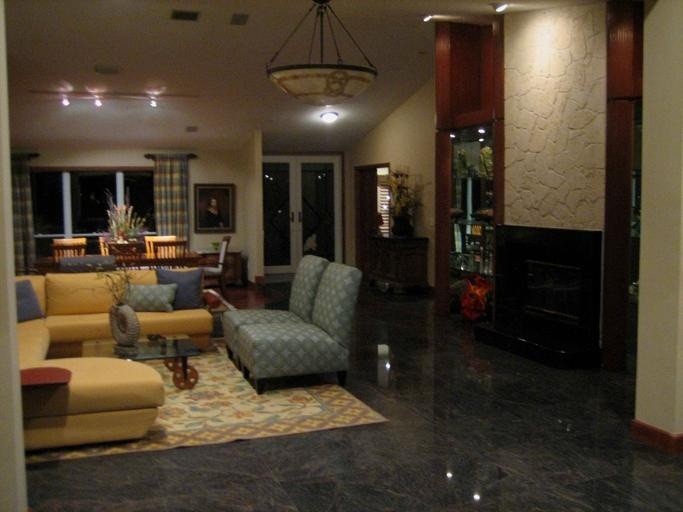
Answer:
[449,130,493,274]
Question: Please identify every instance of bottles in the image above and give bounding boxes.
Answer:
[461,224,494,277]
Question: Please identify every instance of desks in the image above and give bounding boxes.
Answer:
[51,252,200,266]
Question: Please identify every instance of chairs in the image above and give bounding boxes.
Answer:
[154,240,190,261]
[197,236,231,292]
[49,244,89,260]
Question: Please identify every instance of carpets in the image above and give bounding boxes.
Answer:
[202,286,237,309]
[23,334,393,469]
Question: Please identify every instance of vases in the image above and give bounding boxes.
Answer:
[392,214,416,238]
[109,242,142,256]
[96,300,146,347]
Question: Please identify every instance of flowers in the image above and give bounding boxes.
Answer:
[107,200,146,245]
[382,165,429,218]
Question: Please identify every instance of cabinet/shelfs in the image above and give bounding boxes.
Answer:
[373,238,431,300]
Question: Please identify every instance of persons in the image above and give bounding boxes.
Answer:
[205,198,224,228]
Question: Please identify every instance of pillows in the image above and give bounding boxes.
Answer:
[121,279,179,316]
[16,278,42,322]
[151,271,204,306]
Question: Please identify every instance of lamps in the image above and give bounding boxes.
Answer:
[258,1,378,107]
[320,112,337,123]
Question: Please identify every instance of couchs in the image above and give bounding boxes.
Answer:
[240,263,363,395]
[219,254,330,356]
[14,277,48,358]
[23,357,165,446]
[46,269,215,346]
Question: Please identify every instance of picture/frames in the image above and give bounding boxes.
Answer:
[193,184,234,234]
